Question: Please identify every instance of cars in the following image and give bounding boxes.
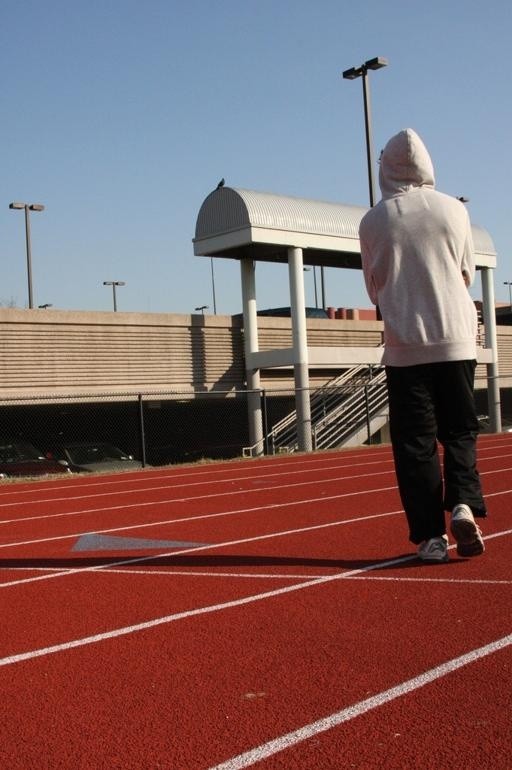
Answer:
[0,434,247,478]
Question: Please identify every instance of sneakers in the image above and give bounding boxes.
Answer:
[418,533,449,560]
[449,503,486,558]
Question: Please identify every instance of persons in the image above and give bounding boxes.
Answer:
[356,126,487,565]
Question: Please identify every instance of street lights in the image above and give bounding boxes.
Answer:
[455,195,469,202]
[343,57,388,206]
[103,281,125,311]
[9,201,53,310]
[195,305,209,315]
[503,280,512,315]
